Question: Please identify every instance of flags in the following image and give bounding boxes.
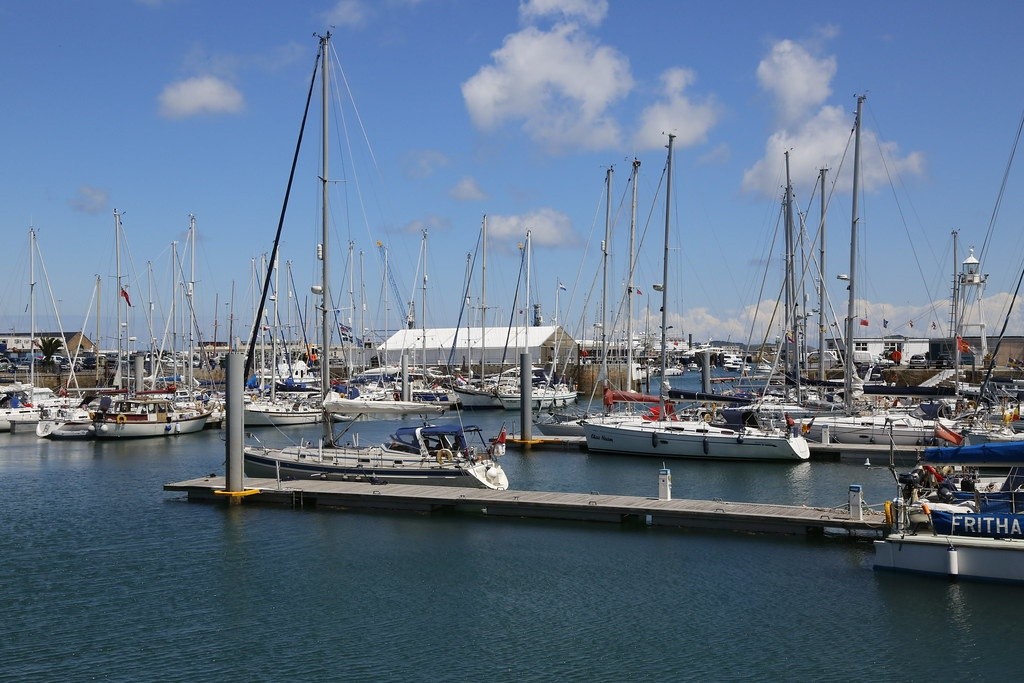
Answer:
[819,327,826,334]
[884,319,888,328]
[496,425,507,444]
[802,415,815,434]
[559,283,566,291]
[121,286,131,307]
[860,319,868,326]
[339,322,362,344]
[627,286,632,294]
[932,322,936,329]
[958,335,969,353]
[785,331,795,343]
[910,320,914,327]
[637,289,642,295]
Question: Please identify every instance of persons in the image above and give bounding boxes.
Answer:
[1005,403,1017,434]
[674,340,678,349]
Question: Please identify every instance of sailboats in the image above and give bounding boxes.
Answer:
[0,27,1024,589]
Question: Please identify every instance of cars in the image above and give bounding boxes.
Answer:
[935,353,955,369]
[909,354,930,369]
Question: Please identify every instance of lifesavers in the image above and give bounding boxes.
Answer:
[252,395,256,401]
[884,500,893,526]
[436,448,453,464]
[116,414,127,424]
